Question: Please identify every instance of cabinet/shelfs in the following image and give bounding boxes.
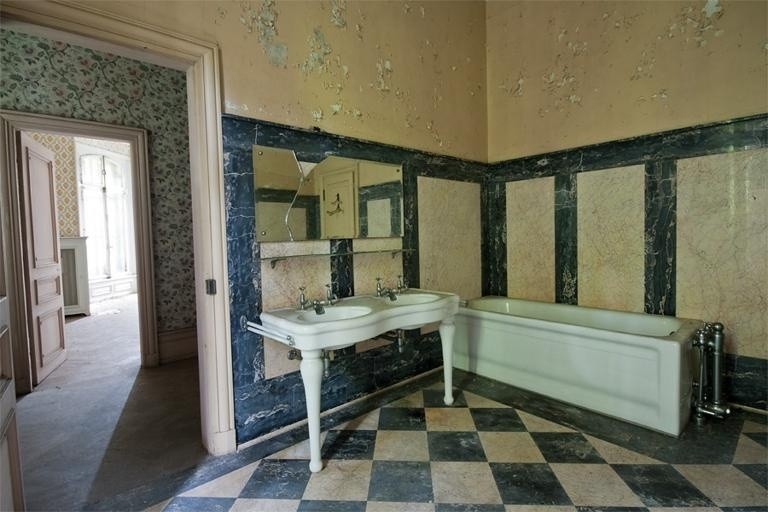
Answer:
[61,236,91,320]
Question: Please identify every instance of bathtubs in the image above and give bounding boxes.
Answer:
[451,295,708,438]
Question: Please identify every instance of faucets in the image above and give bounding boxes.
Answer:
[459,299,470,308]
[312,300,324,314]
[385,289,398,301]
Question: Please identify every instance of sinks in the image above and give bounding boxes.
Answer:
[385,293,440,305]
[297,305,372,322]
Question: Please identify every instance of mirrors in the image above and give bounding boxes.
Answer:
[254,144,406,242]
